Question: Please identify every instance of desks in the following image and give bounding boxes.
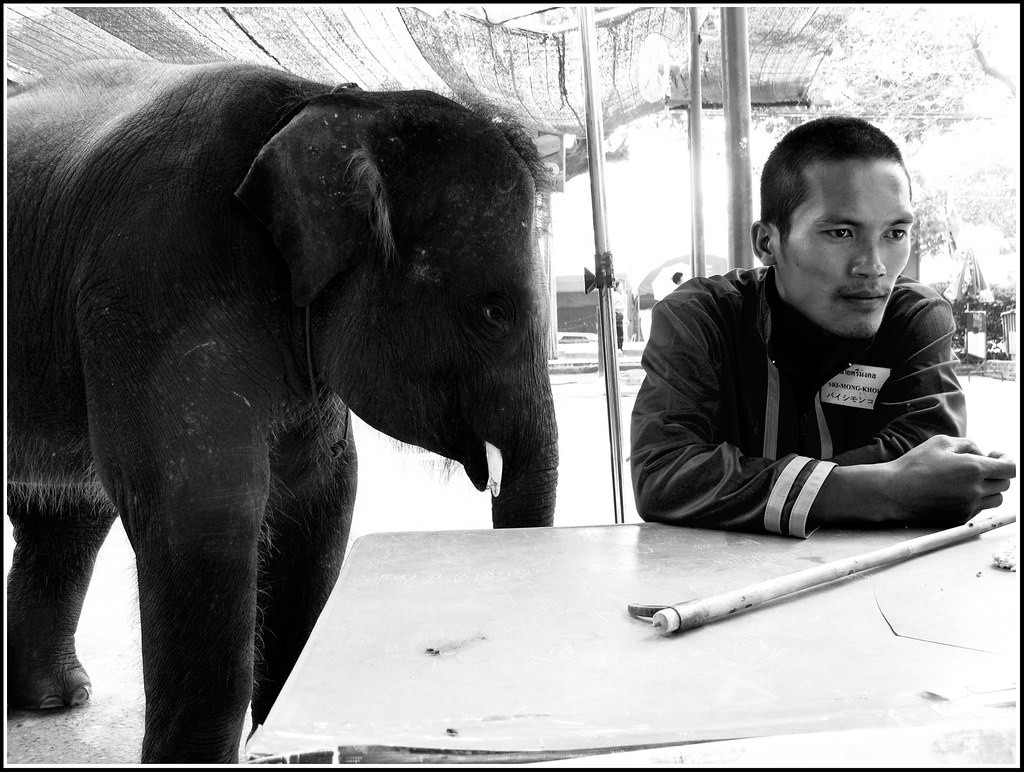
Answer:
[244,518,1020,768]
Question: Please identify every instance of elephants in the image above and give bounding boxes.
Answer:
[7,50,557,766]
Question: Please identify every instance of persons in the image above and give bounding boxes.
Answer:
[630,116,1016,539]
[613,282,626,356]
[673,272,684,289]
